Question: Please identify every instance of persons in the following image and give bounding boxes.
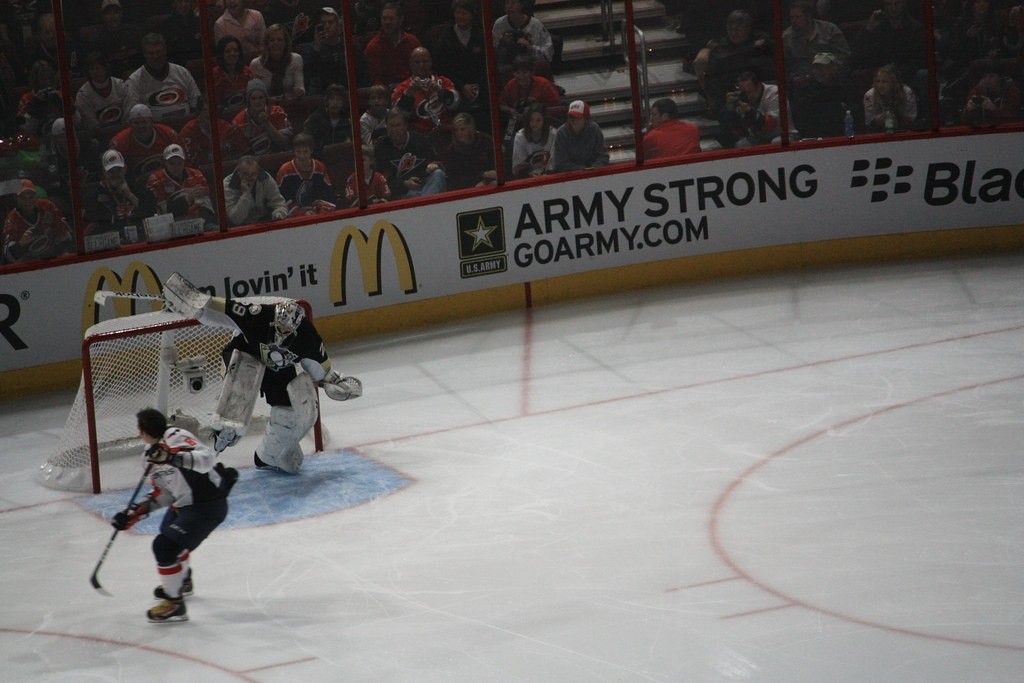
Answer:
[0,0,1024,270]
[111,408,229,624]
[161,273,362,476]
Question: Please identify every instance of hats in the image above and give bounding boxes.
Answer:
[101,149,125,172]
[101,0,122,12]
[314,6,340,20]
[17,179,36,197]
[812,52,837,66]
[52,118,68,136]
[163,143,186,162]
[566,100,590,119]
[246,78,272,119]
[128,104,154,121]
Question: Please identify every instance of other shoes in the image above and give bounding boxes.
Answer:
[214,427,237,458]
[254,450,283,472]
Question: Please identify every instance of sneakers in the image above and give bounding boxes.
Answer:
[153,568,194,599]
[145,597,188,623]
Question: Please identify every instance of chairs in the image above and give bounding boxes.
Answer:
[71,0,353,179]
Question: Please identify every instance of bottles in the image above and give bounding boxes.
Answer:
[844,110,855,139]
[884,109,894,133]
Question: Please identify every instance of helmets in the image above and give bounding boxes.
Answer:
[274,301,305,336]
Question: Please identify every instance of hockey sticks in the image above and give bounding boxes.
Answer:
[90,463,155,597]
[93,290,166,307]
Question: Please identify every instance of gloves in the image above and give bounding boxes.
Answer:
[145,443,180,464]
[111,504,148,530]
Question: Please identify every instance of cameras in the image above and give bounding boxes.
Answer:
[419,80,425,86]
[971,95,984,105]
[736,92,749,103]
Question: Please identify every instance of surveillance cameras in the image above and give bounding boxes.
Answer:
[183,371,205,395]
